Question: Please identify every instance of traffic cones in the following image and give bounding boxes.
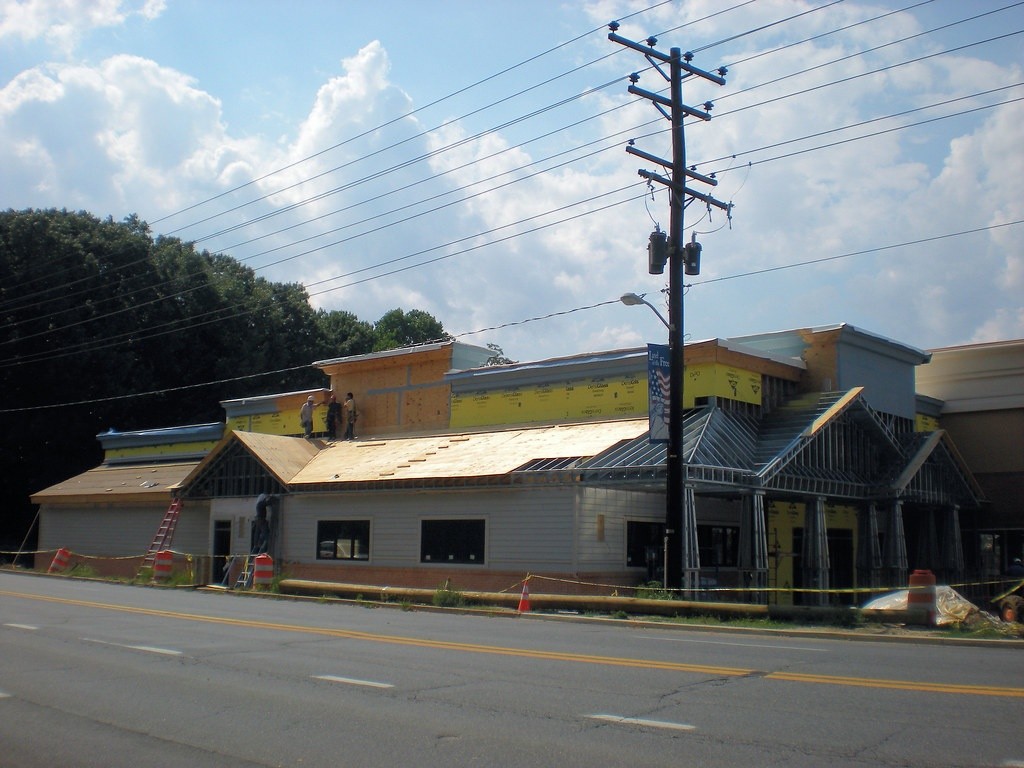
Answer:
[517,580,532,613]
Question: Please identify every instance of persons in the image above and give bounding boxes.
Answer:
[254,495,274,551]
[327,395,342,441]
[1006,558,1024,597]
[300,396,326,439]
[344,393,356,440]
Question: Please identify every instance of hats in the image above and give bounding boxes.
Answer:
[307,396,314,400]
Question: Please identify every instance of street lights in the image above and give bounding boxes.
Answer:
[619,292,682,602]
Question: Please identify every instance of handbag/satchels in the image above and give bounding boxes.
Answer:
[299,420,305,427]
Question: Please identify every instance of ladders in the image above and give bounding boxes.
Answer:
[137,495,183,577]
